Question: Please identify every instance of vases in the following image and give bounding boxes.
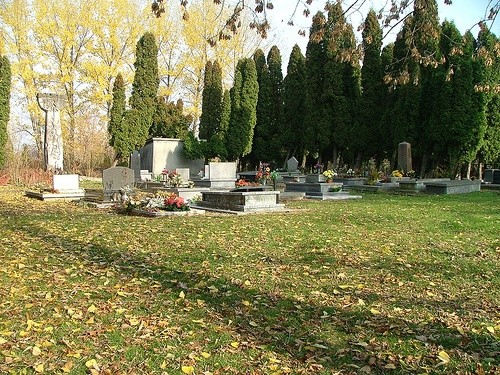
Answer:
[326,178,333,183]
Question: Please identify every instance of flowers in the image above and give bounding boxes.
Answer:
[323,169,338,179]
